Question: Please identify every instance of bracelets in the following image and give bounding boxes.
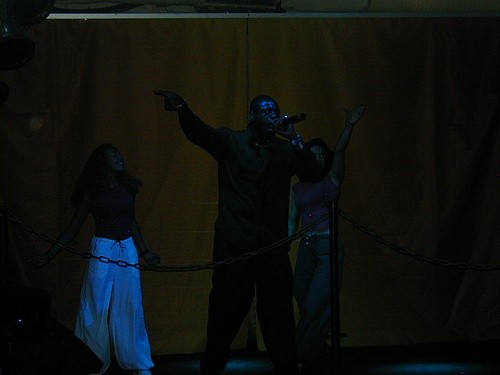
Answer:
[346,119,355,128]
[289,133,304,152]
[175,101,187,111]
[46,253,49,264]
[141,249,148,256]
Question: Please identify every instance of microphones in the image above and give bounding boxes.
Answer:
[269,112,306,131]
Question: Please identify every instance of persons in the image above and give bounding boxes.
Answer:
[152,89,321,375]
[285,104,366,374]
[29,143,181,375]
